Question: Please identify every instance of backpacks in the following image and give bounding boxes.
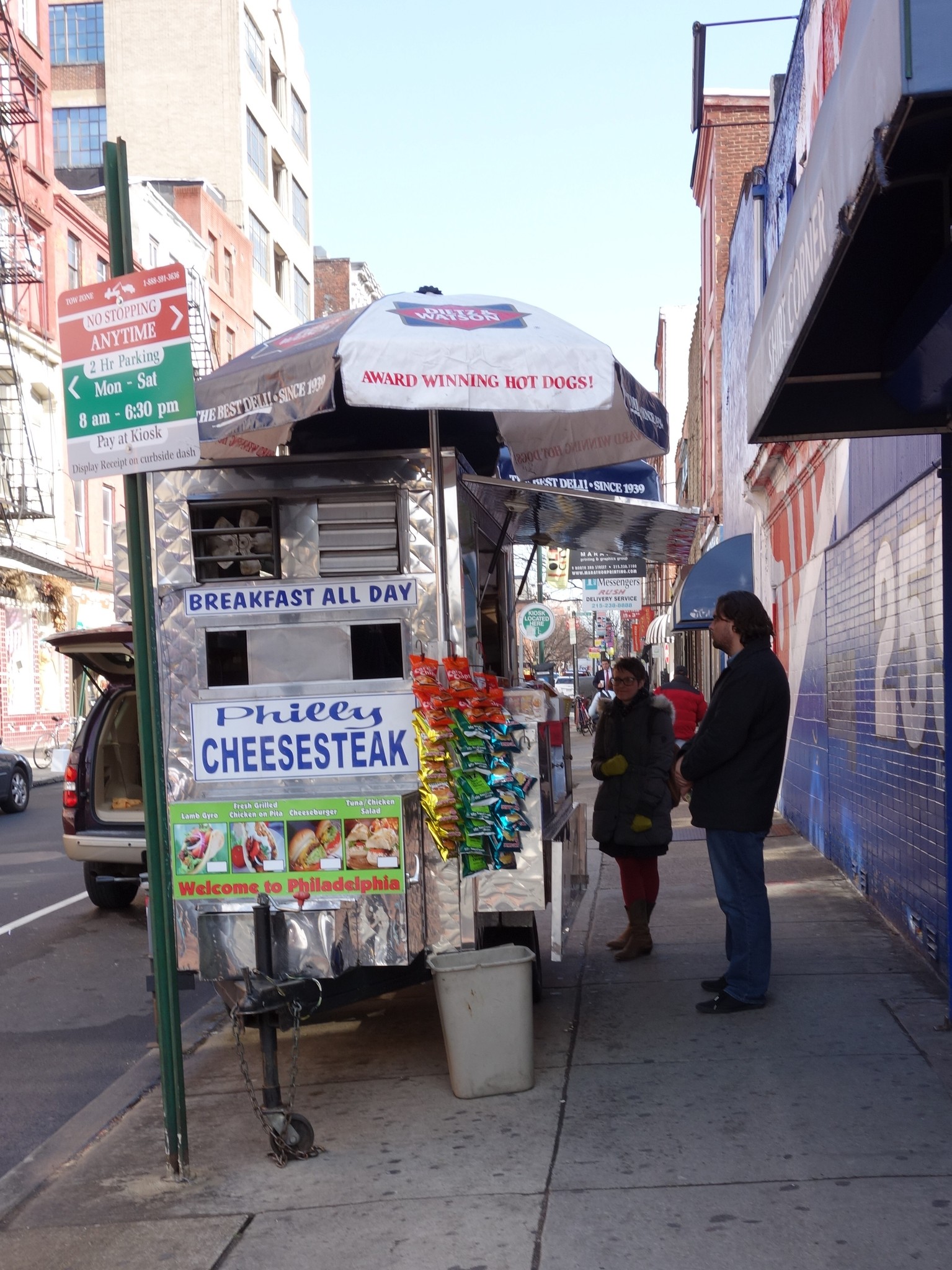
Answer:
[669,742,683,807]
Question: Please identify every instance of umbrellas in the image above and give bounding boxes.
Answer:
[194,287,670,638]
[494,444,660,501]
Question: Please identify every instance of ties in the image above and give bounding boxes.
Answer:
[606,670,609,690]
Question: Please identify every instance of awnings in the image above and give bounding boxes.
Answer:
[646,614,674,644]
[747,0,952,442]
[675,534,752,623]
[460,473,701,624]
[671,591,712,632]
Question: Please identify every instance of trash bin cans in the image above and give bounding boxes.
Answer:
[426,945,535,1099]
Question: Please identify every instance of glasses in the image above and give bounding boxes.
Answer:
[610,677,634,686]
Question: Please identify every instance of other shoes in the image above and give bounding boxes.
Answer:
[695,995,764,1013]
[700,977,728,991]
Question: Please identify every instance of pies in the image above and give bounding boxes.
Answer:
[232,821,279,873]
[179,828,225,874]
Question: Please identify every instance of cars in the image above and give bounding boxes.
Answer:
[0,737,34,813]
[536,670,598,708]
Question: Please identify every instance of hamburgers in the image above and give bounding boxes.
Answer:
[347,817,399,867]
[315,821,341,853]
[288,828,327,871]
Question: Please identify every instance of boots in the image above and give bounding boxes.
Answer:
[613,902,653,960]
[605,900,656,949]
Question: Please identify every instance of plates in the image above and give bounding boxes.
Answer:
[242,827,286,873]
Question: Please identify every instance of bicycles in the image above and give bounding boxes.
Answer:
[33,715,86,769]
[568,692,594,736]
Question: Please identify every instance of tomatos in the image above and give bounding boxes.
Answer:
[231,846,246,867]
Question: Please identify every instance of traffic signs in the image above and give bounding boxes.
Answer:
[57,263,201,481]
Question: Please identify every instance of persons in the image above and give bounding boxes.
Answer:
[588,678,616,719]
[593,658,613,691]
[675,590,790,1013]
[656,665,707,747]
[590,656,675,961]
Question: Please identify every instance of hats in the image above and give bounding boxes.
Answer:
[675,666,687,675]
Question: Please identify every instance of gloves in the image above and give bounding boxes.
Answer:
[631,813,652,832]
[600,754,628,776]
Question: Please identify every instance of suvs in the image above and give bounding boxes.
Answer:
[40,621,147,909]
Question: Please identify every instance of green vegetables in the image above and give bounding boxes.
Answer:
[178,823,213,872]
